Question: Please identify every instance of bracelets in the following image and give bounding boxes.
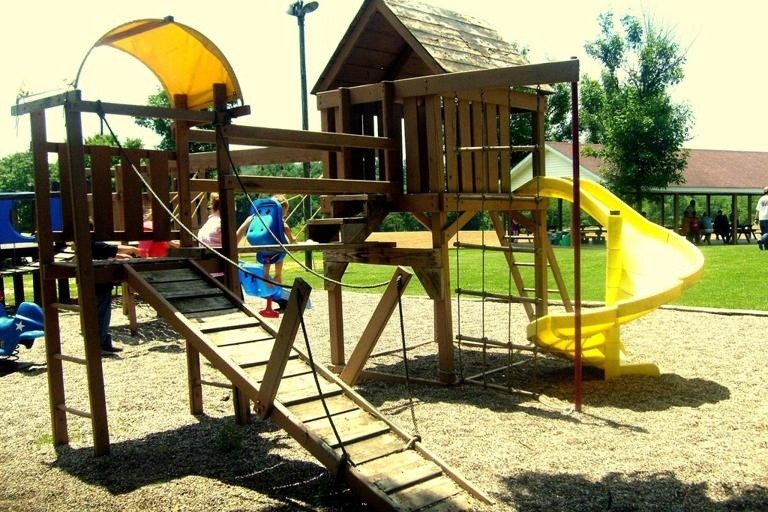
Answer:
[755,219,759,220]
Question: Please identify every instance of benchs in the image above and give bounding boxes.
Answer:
[577,224,760,244]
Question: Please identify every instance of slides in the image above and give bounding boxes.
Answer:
[515,179,705,379]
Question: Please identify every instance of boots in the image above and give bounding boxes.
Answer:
[99,334,122,356]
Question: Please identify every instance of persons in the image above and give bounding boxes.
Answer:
[197,189,254,302]
[755,186,768,250]
[134,205,180,259]
[684,199,696,240]
[713,209,729,240]
[686,209,700,242]
[510,217,520,244]
[640,211,647,218]
[699,212,712,245]
[70,218,143,358]
[751,220,762,241]
[262,192,298,289]
